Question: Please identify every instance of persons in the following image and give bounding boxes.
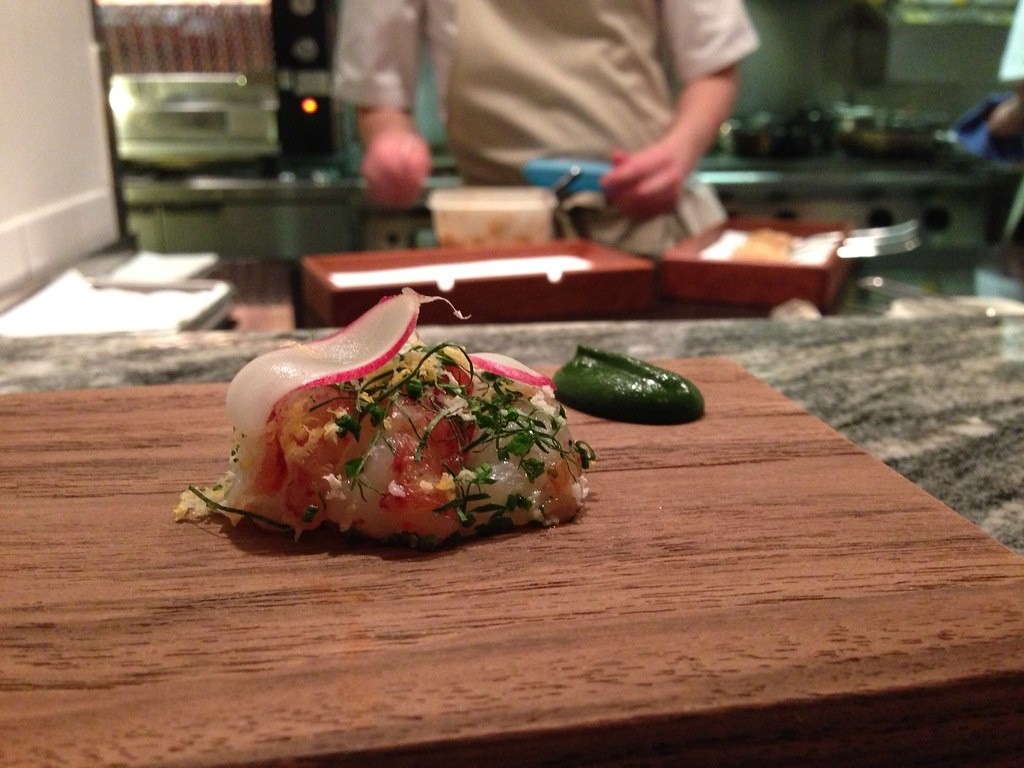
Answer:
[337,0,761,255]
[988,0,1024,161]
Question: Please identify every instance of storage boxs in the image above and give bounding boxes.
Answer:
[299,241,662,327]
[660,215,874,313]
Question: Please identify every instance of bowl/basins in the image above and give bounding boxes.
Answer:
[427,187,556,252]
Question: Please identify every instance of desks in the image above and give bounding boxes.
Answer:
[2,355,1023,768]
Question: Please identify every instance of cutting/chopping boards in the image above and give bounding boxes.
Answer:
[0,356,1024,768]
